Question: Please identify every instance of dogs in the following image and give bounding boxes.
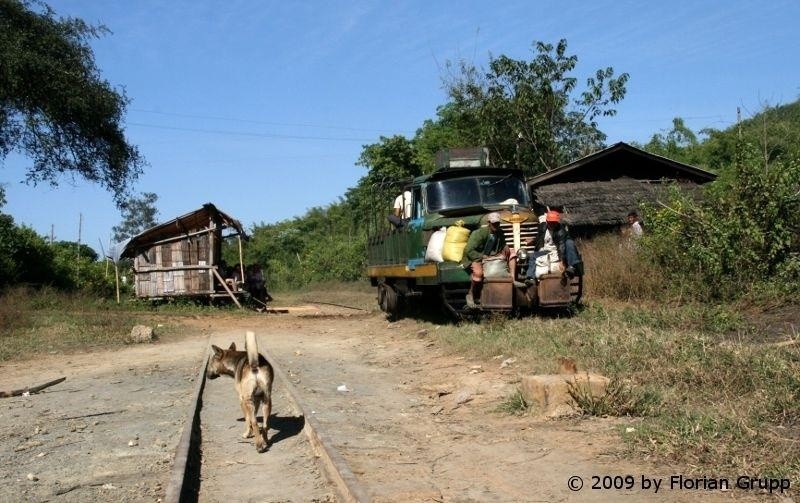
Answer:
[206,331,274,453]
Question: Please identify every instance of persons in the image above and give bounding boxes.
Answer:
[388,180,414,230]
[463,211,526,309]
[220,259,276,304]
[627,211,643,250]
[524,210,581,285]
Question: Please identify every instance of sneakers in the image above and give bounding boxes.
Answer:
[514,282,526,287]
[565,268,574,277]
[525,280,535,285]
[466,295,477,309]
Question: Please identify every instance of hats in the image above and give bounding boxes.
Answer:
[547,211,560,222]
[488,213,501,224]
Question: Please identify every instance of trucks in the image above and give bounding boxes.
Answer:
[366,147,585,312]
